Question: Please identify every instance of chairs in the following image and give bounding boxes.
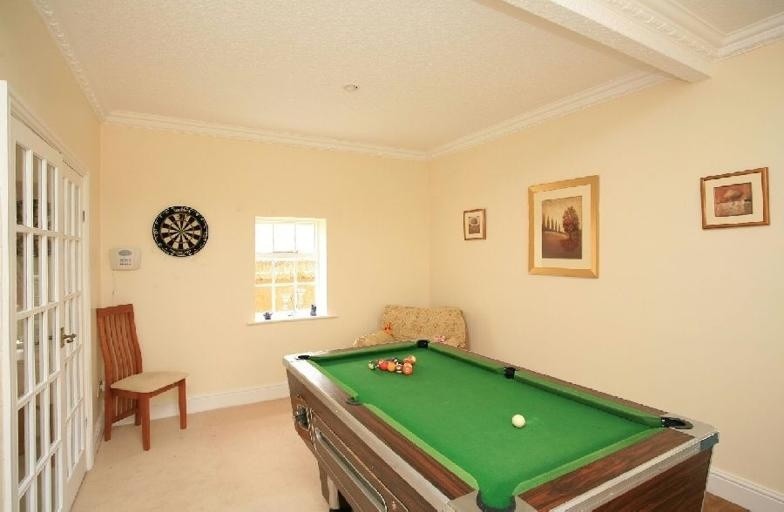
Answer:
[95,303,188,451]
[355,305,467,350]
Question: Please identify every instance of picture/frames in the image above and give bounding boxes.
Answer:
[463,208,486,242]
[526,174,603,281]
[699,165,771,231]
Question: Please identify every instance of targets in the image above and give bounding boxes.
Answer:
[152,206,209,257]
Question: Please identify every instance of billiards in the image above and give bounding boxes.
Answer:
[512,414,525,428]
[369,355,416,375]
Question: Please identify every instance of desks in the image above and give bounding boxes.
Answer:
[281,339,720,512]
